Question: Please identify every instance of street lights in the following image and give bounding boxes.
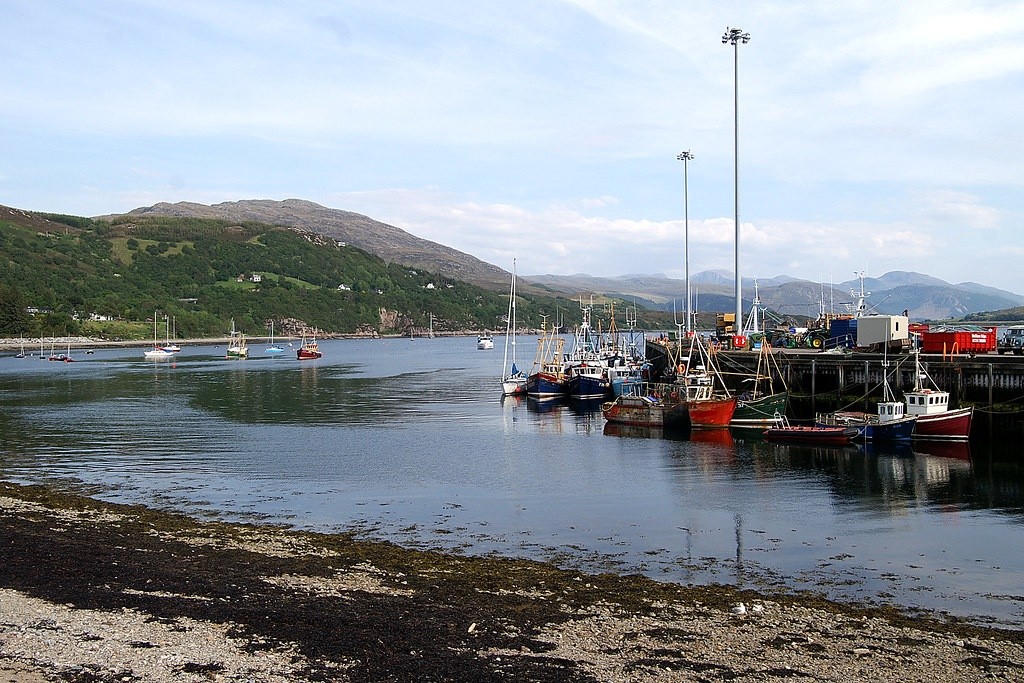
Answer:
[675,148,694,341]
[720,25,751,351]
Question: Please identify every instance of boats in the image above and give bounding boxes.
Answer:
[726,333,789,431]
[602,331,738,431]
[49,332,64,361]
[478,334,494,350]
[814,410,920,445]
[39,332,46,358]
[811,346,976,443]
[765,412,862,445]
[63,331,74,363]
[500,258,652,414]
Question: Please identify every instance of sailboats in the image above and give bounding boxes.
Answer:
[143,310,174,356]
[225,321,250,360]
[264,321,284,353]
[157,315,181,353]
[296,327,323,360]
[15,332,27,358]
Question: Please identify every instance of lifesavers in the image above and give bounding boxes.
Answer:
[920,390,931,393]
[678,364,685,373]
